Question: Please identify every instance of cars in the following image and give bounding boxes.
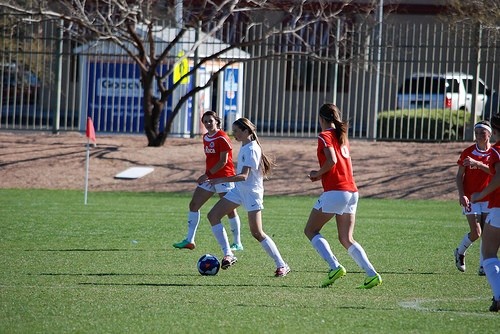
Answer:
[0,68,43,105]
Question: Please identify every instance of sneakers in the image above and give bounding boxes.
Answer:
[172,239,196,250]
[274,262,291,278]
[221,256,237,269]
[355,273,382,290]
[453,247,466,272]
[230,242,244,251]
[319,265,347,288]
[478,266,485,277]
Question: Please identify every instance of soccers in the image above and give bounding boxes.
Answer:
[197,253,220,276]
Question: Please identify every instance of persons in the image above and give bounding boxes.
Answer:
[206,117,291,277]
[304,104,382,289]
[454,120,494,275]
[172,110,244,251]
[472,114,500,312]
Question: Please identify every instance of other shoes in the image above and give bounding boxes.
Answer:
[489,296,500,313]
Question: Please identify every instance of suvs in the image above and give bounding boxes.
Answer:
[396,71,496,117]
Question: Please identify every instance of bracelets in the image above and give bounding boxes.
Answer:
[205,171,212,177]
